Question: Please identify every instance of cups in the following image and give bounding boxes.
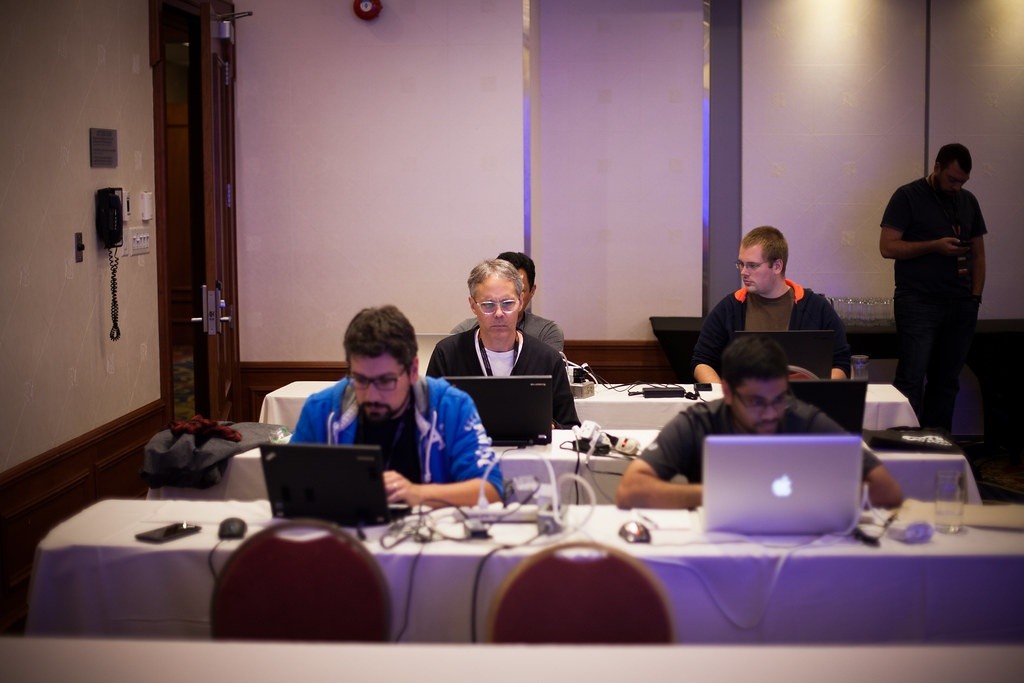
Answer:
[824,296,895,327]
[933,469,965,535]
[850,355,870,383]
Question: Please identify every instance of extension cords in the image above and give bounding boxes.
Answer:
[538,505,569,535]
[453,503,539,522]
[598,433,640,455]
[570,382,594,399]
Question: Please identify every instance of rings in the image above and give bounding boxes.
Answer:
[393,483,399,490]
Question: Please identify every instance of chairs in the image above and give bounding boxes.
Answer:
[484,542,673,644]
[210,520,391,641]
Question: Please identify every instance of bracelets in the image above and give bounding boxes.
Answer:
[972,294,983,304]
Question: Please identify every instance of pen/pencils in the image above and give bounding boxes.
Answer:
[637,512,658,528]
[884,512,896,528]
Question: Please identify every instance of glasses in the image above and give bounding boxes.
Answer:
[734,259,773,272]
[346,361,408,391]
[730,386,795,409]
[471,294,521,315]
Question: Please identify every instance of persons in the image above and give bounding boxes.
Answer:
[425,258,582,430]
[615,334,904,511]
[868,142,988,451]
[448,251,563,352]
[287,303,504,509]
[690,226,852,383]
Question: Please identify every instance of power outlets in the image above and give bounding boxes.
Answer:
[120,228,129,256]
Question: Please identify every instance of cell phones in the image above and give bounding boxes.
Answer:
[694,383,712,391]
[135,523,200,543]
[958,241,974,247]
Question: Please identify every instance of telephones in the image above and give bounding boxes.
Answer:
[96,187,123,245]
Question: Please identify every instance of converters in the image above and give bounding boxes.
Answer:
[642,387,684,398]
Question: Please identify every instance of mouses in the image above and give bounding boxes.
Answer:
[217,517,247,540]
[618,522,651,543]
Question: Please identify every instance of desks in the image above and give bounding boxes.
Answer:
[31,494,1024,642]
[150,427,980,503]
[259,379,919,433]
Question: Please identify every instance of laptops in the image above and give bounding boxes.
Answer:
[701,435,862,535]
[258,441,412,526]
[732,330,832,379]
[441,375,553,446]
[786,380,868,439]
